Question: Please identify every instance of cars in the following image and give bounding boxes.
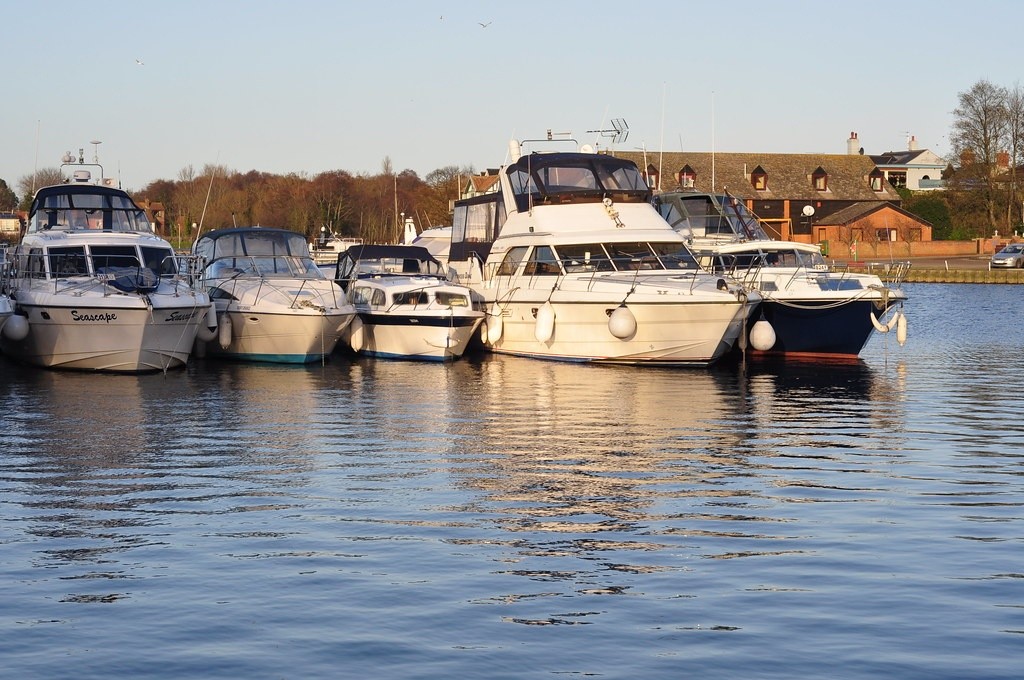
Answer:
[990,244,1024,269]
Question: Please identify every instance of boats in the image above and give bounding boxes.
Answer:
[0,138,214,377]
[306,220,364,268]
[184,169,359,367]
[654,122,912,366]
[400,118,763,370]
[313,244,487,363]
